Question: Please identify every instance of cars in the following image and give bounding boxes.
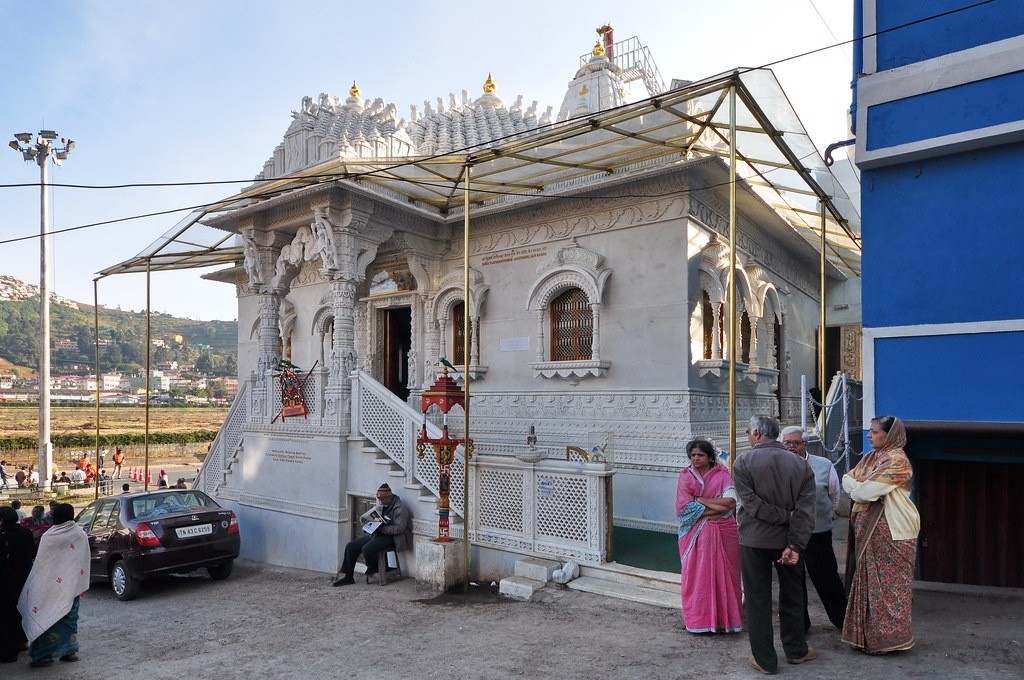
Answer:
[76,488,241,601]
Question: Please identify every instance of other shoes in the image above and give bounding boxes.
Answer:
[749,655,774,674]
[789,647,816,664]
[366,565,377,575]
[332,576,355,586]
[59,653,77,662]
[30,655,52,667]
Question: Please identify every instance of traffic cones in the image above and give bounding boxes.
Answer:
[128,466,153,483]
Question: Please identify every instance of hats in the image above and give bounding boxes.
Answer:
[376,483,393,498]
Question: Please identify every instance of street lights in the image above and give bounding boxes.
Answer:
[10,128,75,497]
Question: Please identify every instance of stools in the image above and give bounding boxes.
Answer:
[364,546,403,586]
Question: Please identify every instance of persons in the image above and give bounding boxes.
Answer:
[733,414,817,674]
[842,416,920,655]
[676,439,743,635]
[332,483,411,588]
[0,447,186,667]
[780,426,849,632]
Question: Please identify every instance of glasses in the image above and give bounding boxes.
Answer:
[782,440,807,448]
